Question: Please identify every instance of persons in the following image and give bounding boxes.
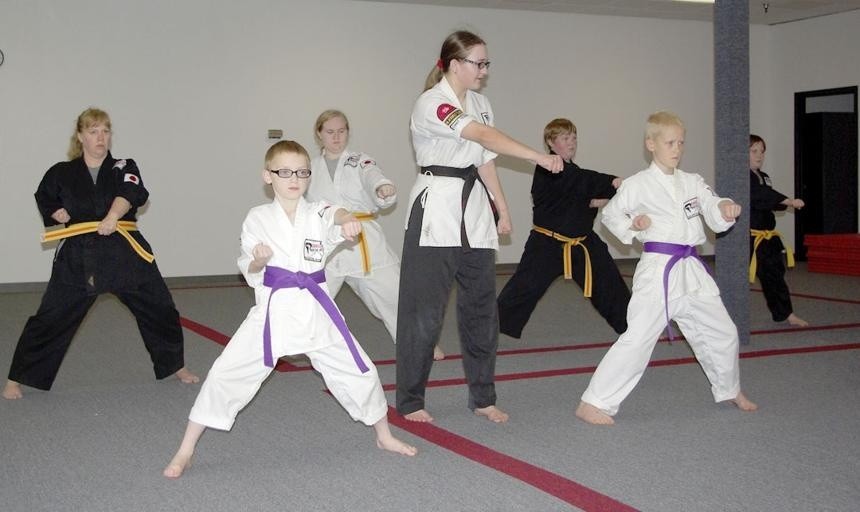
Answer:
[497,119,632,339]
[575,112,759,425]
[288,111,445,361]
[395,31,564,423]
[750,135,810,327]
[2,110,199,400]
[164,140,417,477]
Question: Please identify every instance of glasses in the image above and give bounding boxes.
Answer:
[461,59,490,69]
[270,168,311,178]
